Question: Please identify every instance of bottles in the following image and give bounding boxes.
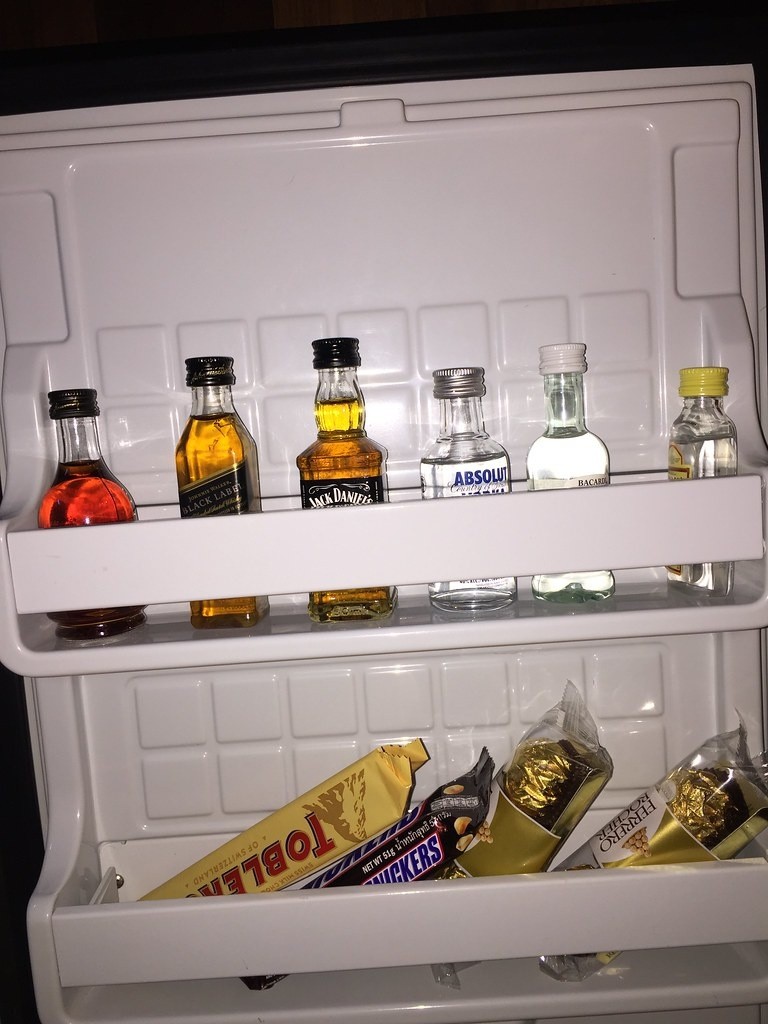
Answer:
[419,367,518,613]
[665,366,739,600]
[35,387,148,640]
[525,342,616,605]
[296,337,398,623]
[174,355,271,629]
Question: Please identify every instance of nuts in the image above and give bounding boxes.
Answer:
[456,835,473,851]
[455,817,472,834]
[443,785,464,794]
